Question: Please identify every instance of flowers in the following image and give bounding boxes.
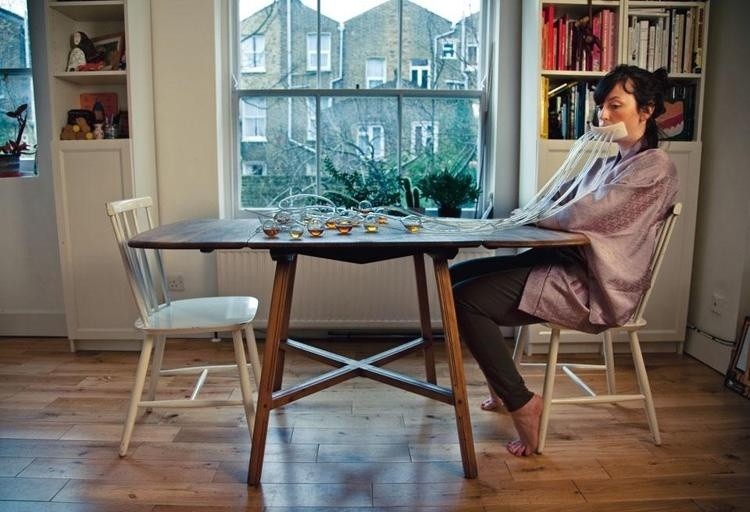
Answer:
[0,104,36,157]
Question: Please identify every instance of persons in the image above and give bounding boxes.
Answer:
[450,66,679,455]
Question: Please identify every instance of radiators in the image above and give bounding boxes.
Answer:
[215,246,496,330]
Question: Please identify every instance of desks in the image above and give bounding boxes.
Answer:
[126,217,588,492]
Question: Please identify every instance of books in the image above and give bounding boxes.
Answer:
[542,1,704,139]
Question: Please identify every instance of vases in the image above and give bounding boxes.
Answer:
[0,153,22,176]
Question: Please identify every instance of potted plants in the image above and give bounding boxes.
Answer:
[417,167,485,217]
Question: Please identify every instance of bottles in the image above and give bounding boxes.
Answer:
[261,199,388,240]
[400,215,423,234]
[94,123,105,140]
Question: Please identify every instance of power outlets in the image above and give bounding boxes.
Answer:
[167,273,186,291]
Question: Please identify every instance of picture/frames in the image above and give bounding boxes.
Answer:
[89,31,125,64]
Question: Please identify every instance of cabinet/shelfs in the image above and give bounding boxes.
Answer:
[520,0,711,360]
[42,0,161,354]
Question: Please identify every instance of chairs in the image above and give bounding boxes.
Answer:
[105,196,263,457]
[513,200,684,457]
